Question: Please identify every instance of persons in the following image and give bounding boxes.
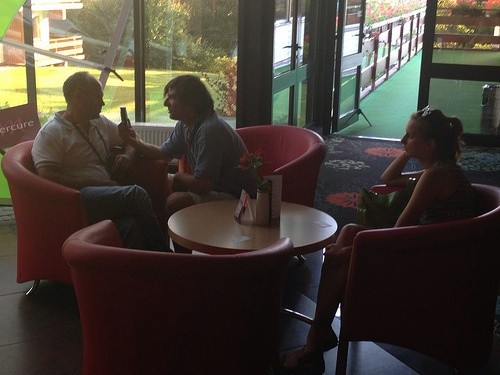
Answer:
[118,75,257,253]
[279,108,476,375]
[31,72,174,254]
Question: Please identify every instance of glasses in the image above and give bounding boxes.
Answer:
[422,105,432,119]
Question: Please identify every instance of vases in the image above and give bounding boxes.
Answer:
[257,175,283,224]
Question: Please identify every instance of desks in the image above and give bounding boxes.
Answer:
[168,198,338,328]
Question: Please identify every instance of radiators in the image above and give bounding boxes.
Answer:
[117,123,179,165]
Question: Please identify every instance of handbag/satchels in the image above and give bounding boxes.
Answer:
[354,187,405,229]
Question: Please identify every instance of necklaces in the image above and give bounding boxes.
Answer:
[65,110,113,180]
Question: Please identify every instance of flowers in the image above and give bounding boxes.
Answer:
[239,151,269,193]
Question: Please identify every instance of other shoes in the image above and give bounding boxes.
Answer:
[280,355,325,375]
[294,328,339,363]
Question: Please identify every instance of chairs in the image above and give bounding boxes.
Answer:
[177,124,326,208]
[335,181,500,375]
[1,138,168,294]
[63,218,293,375]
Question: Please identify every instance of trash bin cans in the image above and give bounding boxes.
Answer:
[480,83,500,136]
